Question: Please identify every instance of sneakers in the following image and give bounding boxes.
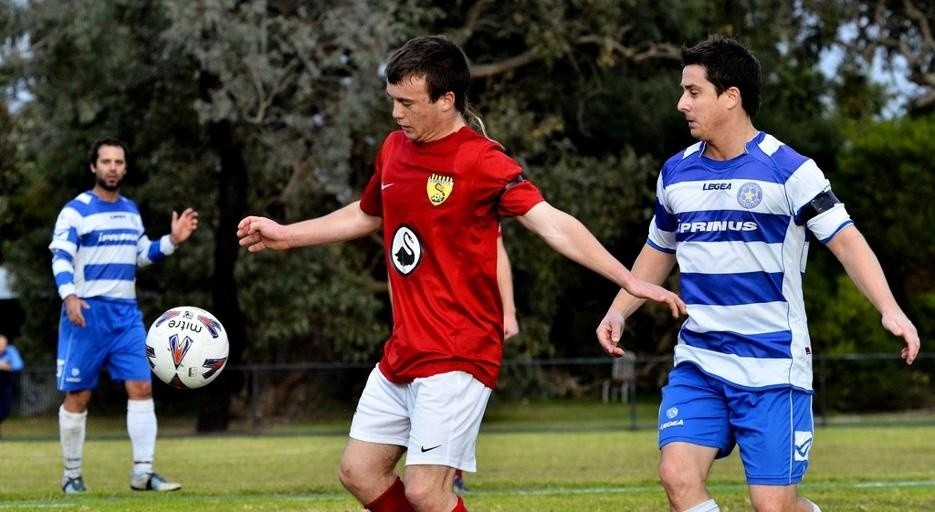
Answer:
[60,474,88,492]
[130,472,181,492]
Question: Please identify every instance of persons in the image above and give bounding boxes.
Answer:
[451,223,519,492]
[0,335,24,421]
[49,137,198,495]
[596,36,920,511]
[237,36,687,512]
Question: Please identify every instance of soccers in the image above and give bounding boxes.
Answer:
[145,307,229,389]
